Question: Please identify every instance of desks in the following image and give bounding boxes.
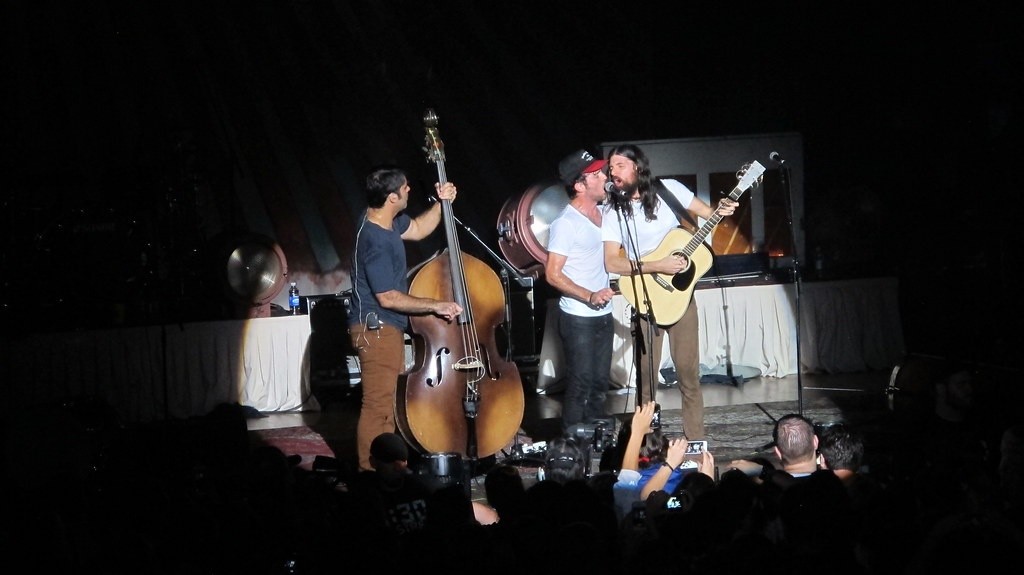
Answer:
[537,271,908,402]
[0,312,311,419]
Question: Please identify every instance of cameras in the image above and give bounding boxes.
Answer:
[566,415,616,476]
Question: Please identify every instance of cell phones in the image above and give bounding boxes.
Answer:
[665,496,684,510]
[650,403,660,430]
[684,440,708,455]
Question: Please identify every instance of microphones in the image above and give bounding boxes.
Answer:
[605,181,627,197]
[770,152,787,165]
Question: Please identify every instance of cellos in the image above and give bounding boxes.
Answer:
[394,105,527,461]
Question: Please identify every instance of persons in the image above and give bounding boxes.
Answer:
[2,401,1022,575]
[545,148,620,431]
[602,144,740,468]
[350,168,463,470]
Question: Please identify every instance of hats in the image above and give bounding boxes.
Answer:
[371,432,408,463]
[558,149,610,186]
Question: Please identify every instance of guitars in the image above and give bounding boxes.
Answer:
[618,157,766,326]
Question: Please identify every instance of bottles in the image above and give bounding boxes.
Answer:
[288,282,301,316]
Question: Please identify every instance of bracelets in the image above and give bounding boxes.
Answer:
[590,292,596,302]
